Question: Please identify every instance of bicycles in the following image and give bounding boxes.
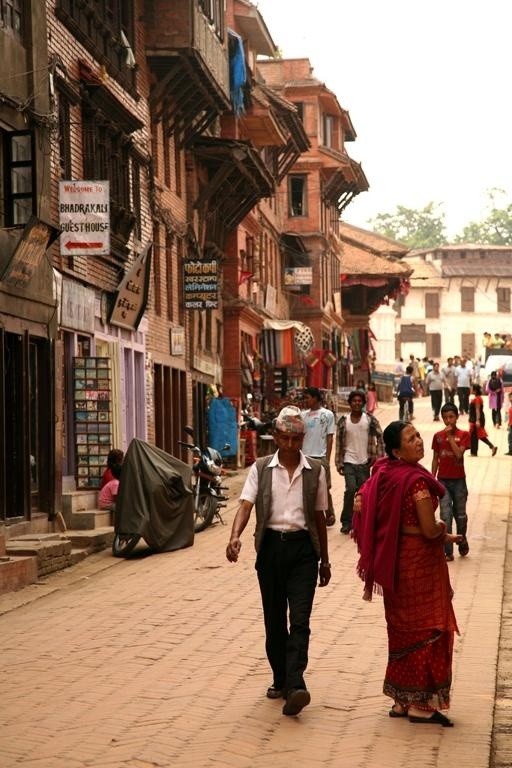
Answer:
[396,395,413,423]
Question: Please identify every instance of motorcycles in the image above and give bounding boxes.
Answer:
[241,392,276,434]
[108,439,195,557]
[175,425,233,532]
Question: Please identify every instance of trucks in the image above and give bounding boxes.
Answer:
[483,348,512,394]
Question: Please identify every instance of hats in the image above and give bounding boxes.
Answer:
[276,405,304,433]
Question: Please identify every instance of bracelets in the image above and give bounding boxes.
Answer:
[319,563,331,568]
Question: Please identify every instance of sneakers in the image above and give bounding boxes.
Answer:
[267,684,282,699]
[282,688,310,714]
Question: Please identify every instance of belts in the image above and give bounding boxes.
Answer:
[267,529,308,541]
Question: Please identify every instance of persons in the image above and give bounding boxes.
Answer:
[98,463,120,513]
[353,420,465,726]
[431,402,471,561]
[226,405,331,716]
[298,387,335,526]
[335,390,385,534]
[99,449,123,491]
[355,331,512,457]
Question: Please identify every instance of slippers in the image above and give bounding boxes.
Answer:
[389,704,451,723]
